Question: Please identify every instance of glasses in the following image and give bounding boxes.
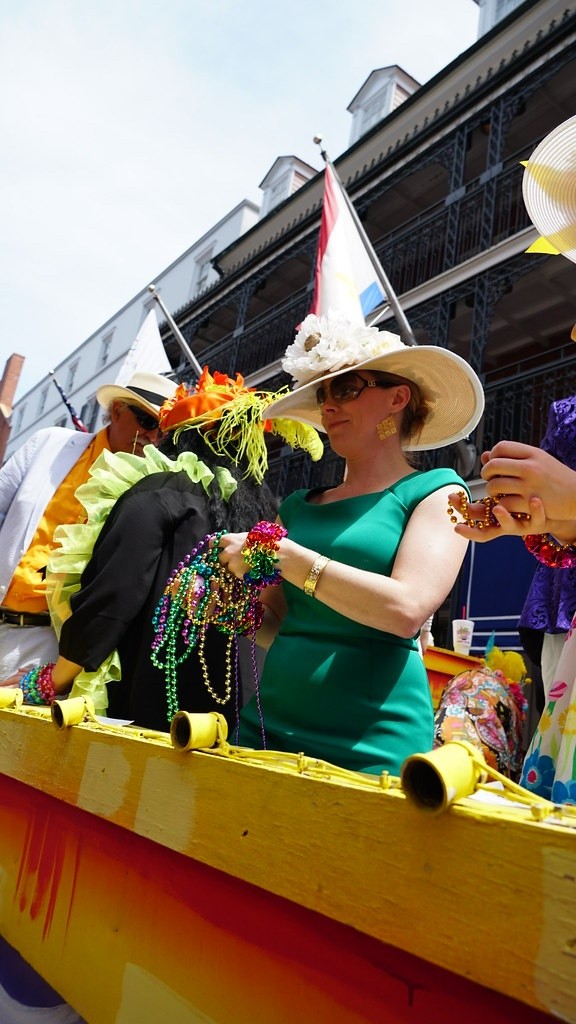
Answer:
[120,401,163,438]
[316,370,405,407]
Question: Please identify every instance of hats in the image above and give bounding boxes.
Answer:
[262,313,486,451]
[96,372,180,421]
[158,366,272,434]
[519,113,576,264]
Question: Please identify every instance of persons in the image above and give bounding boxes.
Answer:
[0,372,180,680]
[450,116,576,805]
[0,365,325,743]
[175,316,486,777]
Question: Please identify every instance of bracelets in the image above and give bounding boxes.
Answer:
[19,662,55,705]
[303,556,330,598]
[241,522,286,590]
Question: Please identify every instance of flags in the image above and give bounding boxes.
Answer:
[312,160,386,323]
[54,378,87,432]
[115,312,171,387]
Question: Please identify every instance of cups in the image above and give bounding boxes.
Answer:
[452,619,475,657]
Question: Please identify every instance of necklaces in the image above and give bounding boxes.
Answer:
[447,491,576,552]
[150,529,266,751]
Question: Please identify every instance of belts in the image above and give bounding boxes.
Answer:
[0,609,51,627]
